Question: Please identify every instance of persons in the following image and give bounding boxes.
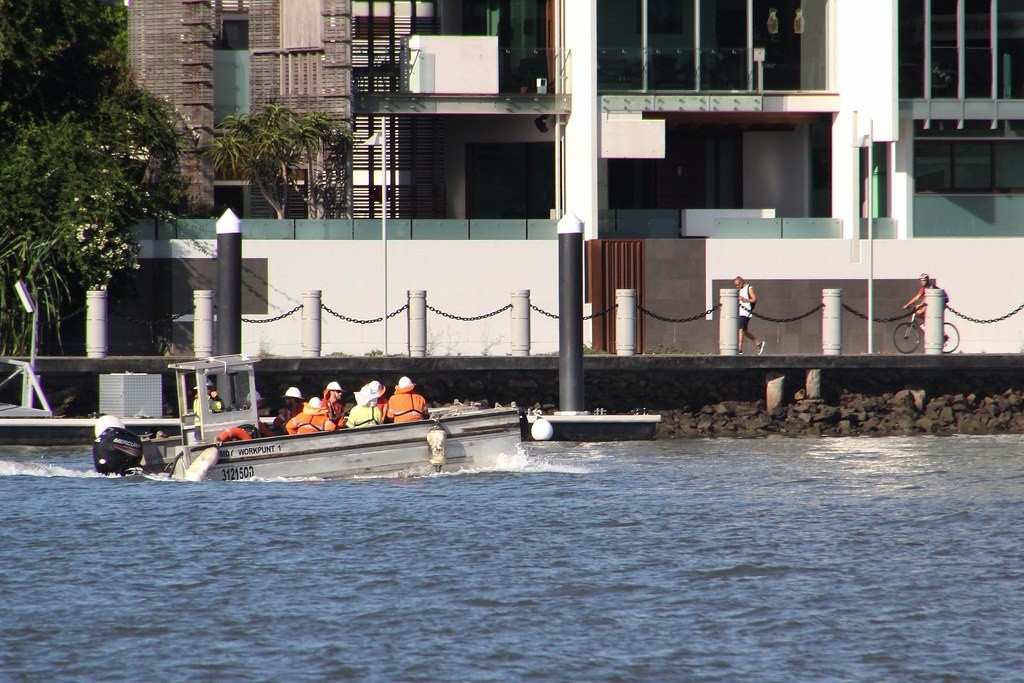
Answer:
[286,397,336,435]
[273,376,428,428]
[193,378,224,441]
[734,276,765,355]
[903,273,947,326]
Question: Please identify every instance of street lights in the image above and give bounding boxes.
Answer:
[366,115,388,350]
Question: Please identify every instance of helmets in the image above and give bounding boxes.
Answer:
[354,380,386,404]
[395,376,415,391]
[246,390,264,402]
[326,382,342,391]
[281,387,306,400]
[302,397,325,410]
[193,378,212,389]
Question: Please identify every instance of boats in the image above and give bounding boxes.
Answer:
[94,354,521,483]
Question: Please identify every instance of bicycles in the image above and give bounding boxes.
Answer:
[893,306,960,354]
